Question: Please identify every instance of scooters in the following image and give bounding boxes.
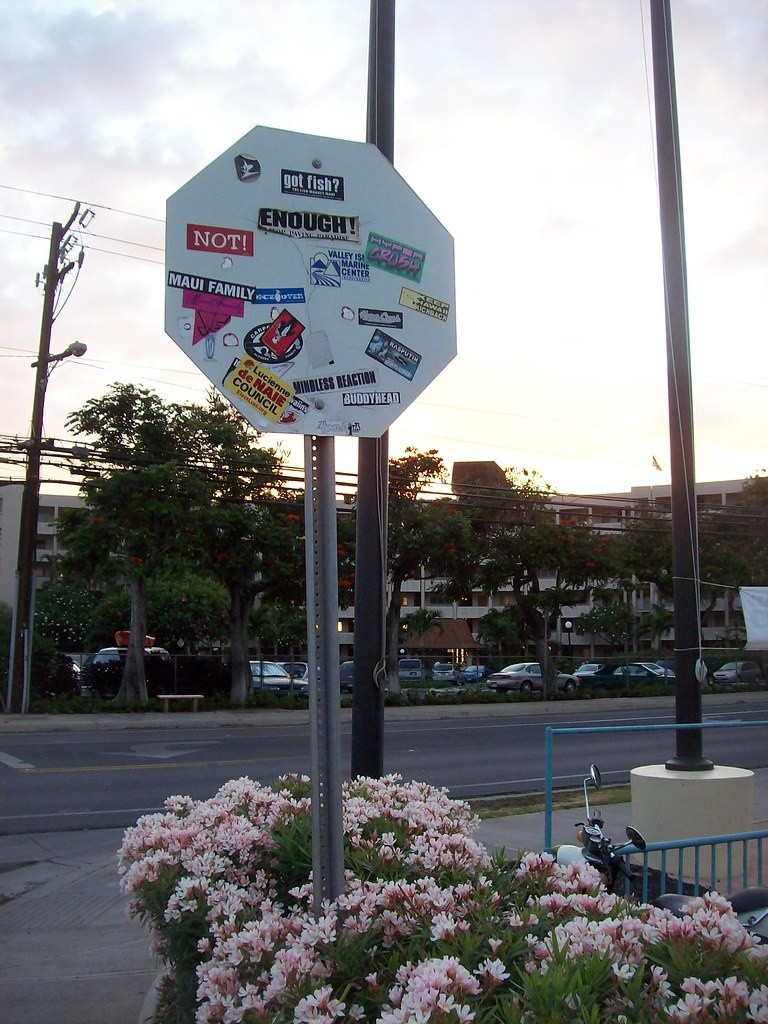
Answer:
[554,763,768,948]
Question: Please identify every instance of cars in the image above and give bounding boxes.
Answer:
[432,661,485,684]
[712,660,763,685]
[572,660,676,686]
[486,662,580,693]
[248,659,353,698]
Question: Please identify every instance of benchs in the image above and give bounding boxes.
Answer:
[157,695,204,712]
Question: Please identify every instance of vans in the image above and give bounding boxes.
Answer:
[397,658,427,682]
[82,647,173,697]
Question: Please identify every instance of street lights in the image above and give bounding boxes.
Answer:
[10,341,87,712]
